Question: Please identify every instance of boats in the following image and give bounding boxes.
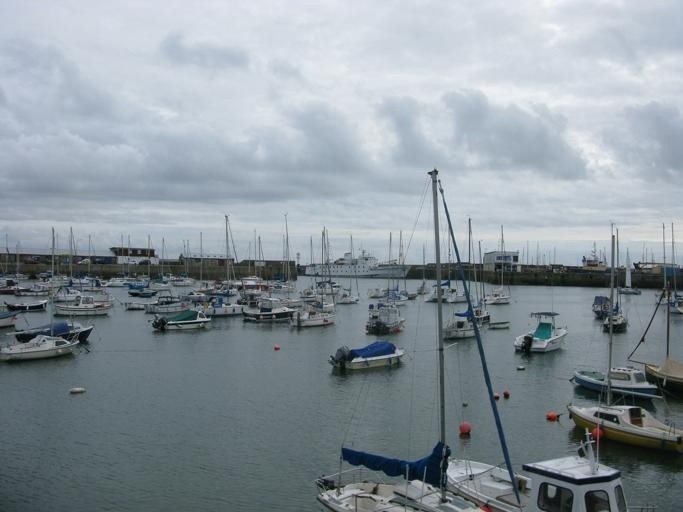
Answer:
[573,366,664,403]
[513,310,568,358]
[327,340,405,377]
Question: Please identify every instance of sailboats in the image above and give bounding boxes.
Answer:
[567,234,683,455]
[365,217,512,341]
[315,169,629,512]
[591,221,641,332]
[625,281,683,401]
[1,213,361,363]
[654,222,683,316]
